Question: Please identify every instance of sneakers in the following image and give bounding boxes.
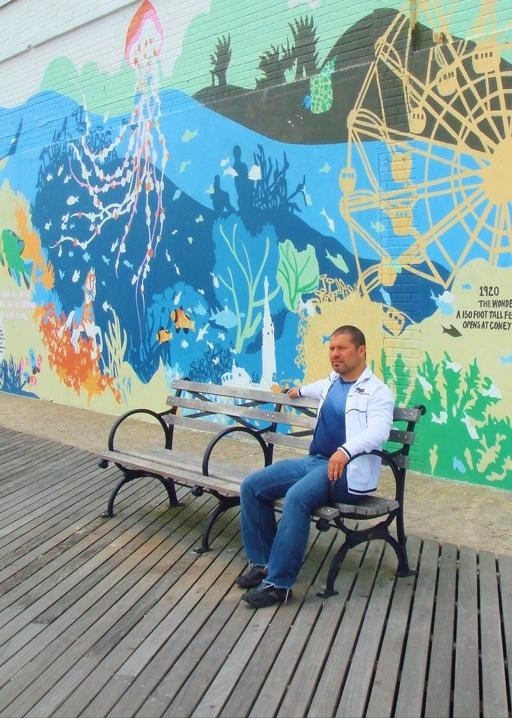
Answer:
[235,565,269,586]
[241,580,292,606]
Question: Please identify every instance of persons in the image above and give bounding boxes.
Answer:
[232,324,398,608]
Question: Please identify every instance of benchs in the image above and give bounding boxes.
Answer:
[101,378,424,598]
